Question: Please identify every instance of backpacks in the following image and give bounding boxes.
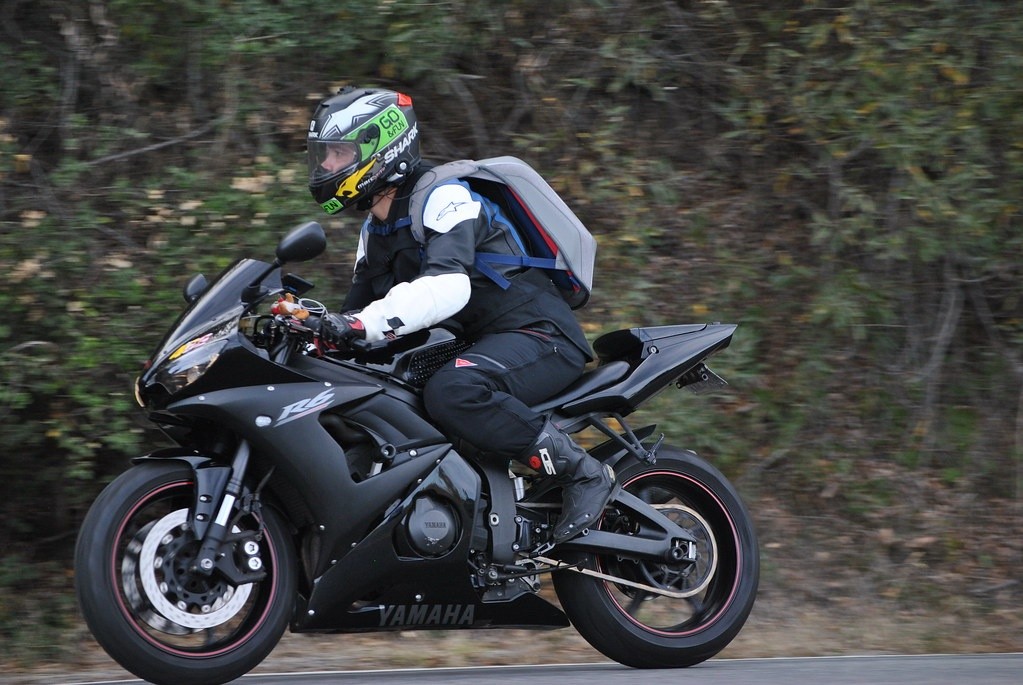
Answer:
[408,155,596,310]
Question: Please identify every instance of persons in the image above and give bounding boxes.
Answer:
[318,95,616,543]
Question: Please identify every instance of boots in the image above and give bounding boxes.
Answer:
[517,415,623,545]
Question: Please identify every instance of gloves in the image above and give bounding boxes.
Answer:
[318,312,366,351]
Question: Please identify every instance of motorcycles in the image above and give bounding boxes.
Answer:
[73,219,762,685]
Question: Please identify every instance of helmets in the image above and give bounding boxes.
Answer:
[306,86,421,215]
